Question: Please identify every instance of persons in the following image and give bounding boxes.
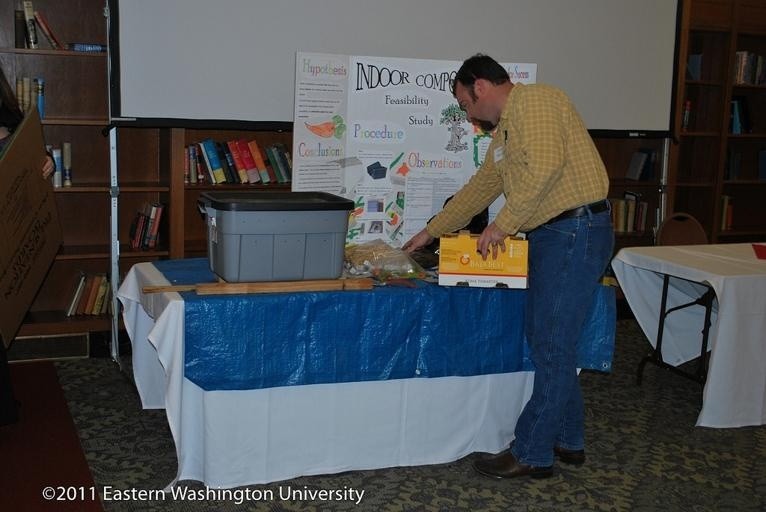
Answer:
[405,54,616,480]
[0,66,57,196]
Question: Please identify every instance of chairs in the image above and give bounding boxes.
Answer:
[653,211,710,246]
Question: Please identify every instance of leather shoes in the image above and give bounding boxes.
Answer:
[555,448,586,463]
[472,448,556,479]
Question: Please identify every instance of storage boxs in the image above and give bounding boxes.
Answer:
[194,187,355,284]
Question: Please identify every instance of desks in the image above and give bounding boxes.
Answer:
[619,241,766,425]
[115,256,540,492]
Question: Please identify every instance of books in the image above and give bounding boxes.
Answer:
[14,76,45,117]
[65,273,111,318]
[18,3,107,52]
[688,49,705,80]
[682,100,693,132]
[617,189,666,233]
[133,203,162,250]
[182,139,291,185]
[631,139,667,185]
[42,142,72,189]
[736,51,763,87]
[732,101,742,132]
[722,195,732,230]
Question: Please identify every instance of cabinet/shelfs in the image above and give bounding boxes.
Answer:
[0,0,766,356]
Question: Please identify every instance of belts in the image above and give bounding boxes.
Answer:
[549,198,611,222]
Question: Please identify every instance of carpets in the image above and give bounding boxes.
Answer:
[50,316,766,512]
